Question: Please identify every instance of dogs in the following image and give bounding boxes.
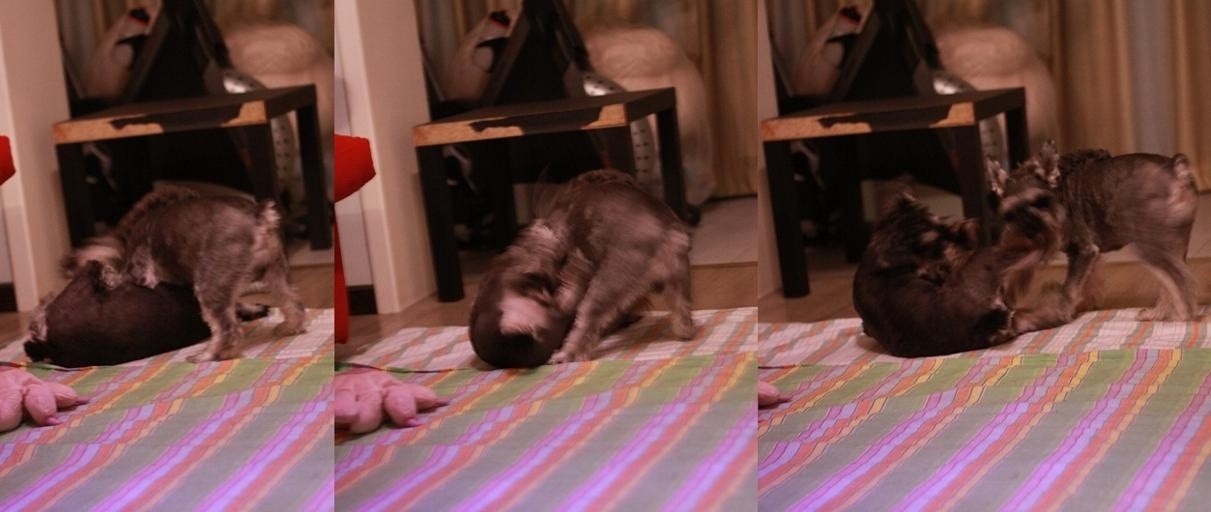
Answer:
[18,179,309,370]
[850,137,1211,364]
[464,165,701,372]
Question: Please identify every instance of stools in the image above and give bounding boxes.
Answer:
[52,82,332,257]
[761,86,1028,298]
[413,87,701,301]
